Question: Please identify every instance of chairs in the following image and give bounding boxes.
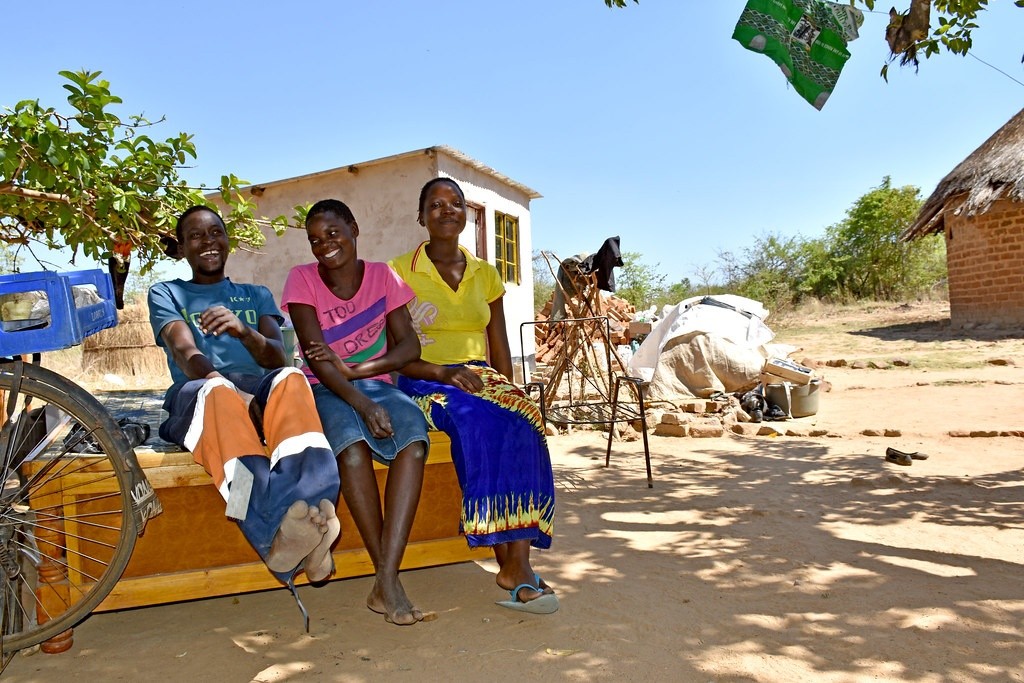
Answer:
[520,316,654,489]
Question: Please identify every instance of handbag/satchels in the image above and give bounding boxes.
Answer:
[64,416,150,454]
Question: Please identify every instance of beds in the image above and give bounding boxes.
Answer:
[24,389,496,654]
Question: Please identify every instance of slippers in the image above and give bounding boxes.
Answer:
[509,574,556,595]
[495,584,559,614]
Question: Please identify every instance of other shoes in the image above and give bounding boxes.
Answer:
[750,409,762,422]
[763,405,786,420]
[911,452,929,460]
[885,447,912,465]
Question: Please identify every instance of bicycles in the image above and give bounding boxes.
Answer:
[0,351,163,679]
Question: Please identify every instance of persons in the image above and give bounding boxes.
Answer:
[384,177,558,615]
[281,196,426,625]
[147,205,342,584]
